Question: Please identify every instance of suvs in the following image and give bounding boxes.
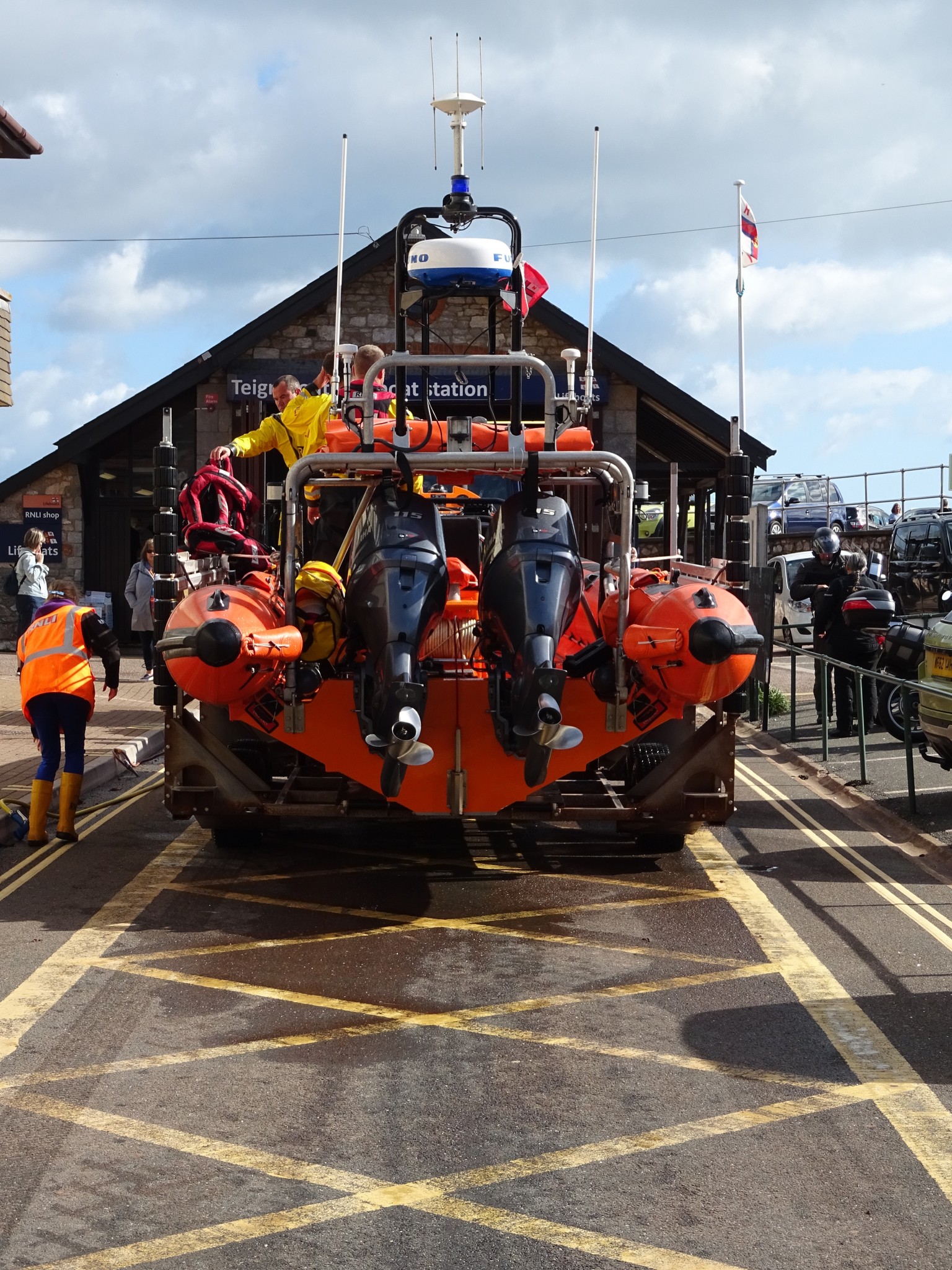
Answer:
[888,512,952,614]
[881,506,952,532]
[845,505,891,531]
[704,473,847,536]
[767,550,856,654]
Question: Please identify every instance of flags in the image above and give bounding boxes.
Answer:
[503,259,549,322]
[741,195,759,268]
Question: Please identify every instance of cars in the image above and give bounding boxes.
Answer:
[636,502,694,537]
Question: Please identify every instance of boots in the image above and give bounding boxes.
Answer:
[55,771,79,842]
[27,779,54,847]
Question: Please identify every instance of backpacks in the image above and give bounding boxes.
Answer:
[3,552,37,597]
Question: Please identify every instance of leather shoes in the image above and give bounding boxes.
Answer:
[816,714,831,724]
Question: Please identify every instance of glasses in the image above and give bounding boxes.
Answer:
[146,549,154,553]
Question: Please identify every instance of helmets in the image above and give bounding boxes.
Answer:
[811,526,841,558]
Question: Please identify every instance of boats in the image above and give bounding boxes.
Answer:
[152,34,765,820]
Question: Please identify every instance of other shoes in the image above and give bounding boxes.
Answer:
[828,727,853,737]
[852,724,869,735]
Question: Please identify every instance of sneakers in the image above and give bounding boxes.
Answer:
[140,674,154,681]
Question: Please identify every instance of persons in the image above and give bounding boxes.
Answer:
[14,527,50,675]
[211,342,414,560]
[17,578,121,847]
[790,527,854,723]
[125,538,154,682]
[815,553,885,738]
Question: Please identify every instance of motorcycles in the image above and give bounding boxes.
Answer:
[842,588,952,742]
